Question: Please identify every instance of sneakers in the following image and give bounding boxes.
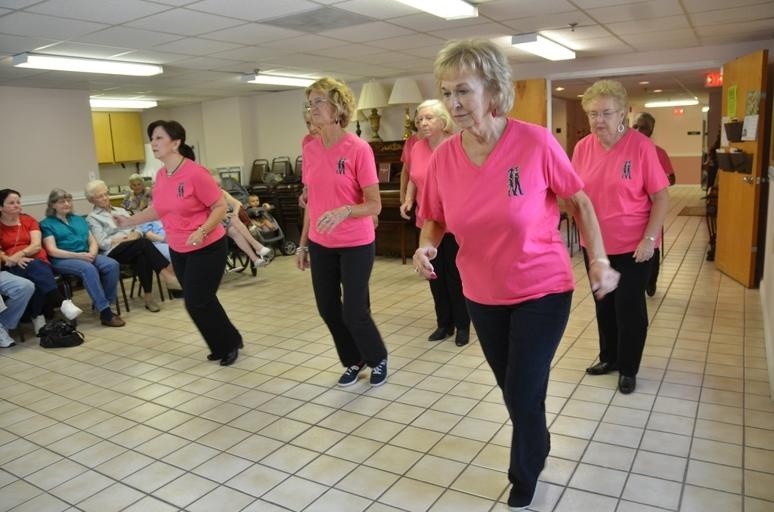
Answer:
[254,258,265,267]
[256,246,271,255]
[507,477,537,510]
[337,361,367,386]
[0,281,182,347]
[370,358,386,386]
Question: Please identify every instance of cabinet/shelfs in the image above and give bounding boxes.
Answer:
[108,194,126,208]
[91,111,145,165]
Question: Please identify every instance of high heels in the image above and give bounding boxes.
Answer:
[429,322,454,340]
[207,352,221,360]
[221,339,243,366]
[456,325,469,346]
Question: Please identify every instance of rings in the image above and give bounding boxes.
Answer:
[413,266,419,273]
[323,217,330,223]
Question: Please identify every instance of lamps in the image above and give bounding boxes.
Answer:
[396,0,478,21]
[11,52,163,77]
[350,77,423,142]
[511,33,576,62]
[241,74,316,87]
[89,100,158,109]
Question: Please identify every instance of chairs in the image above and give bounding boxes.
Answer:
[246,155,304,235]
[18,214,164,342]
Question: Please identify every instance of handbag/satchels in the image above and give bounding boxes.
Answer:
[262,172,278,184]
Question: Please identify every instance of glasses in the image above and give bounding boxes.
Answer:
[585,110,617,118]
[305,96,328,111]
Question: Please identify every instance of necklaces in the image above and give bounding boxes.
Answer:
[171,157,184,175]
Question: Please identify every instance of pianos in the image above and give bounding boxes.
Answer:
[365,141,422,266]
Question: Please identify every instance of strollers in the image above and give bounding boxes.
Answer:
[215,176,295,256]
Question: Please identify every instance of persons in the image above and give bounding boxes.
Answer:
[294,77,388,387]
[413,37,622,509]
[400,99,471,347]
[1,172,277,347]
[112,120,244,366]
[573,78,670,394]
[632,112,675,296]
[399,109,425,230]
[298,101,378,316]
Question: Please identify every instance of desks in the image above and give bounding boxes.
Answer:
[379,190,418,265]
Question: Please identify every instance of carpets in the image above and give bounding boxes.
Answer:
[677,206,707,217]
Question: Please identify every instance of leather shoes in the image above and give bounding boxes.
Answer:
[587,362,620,374]
[646,282,656,296]
[619,372,635,394]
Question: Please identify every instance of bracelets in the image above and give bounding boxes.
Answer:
[587,257,610,265]
[644,234,657,243]
[345,204,354,214]
[294,244,309,254]
